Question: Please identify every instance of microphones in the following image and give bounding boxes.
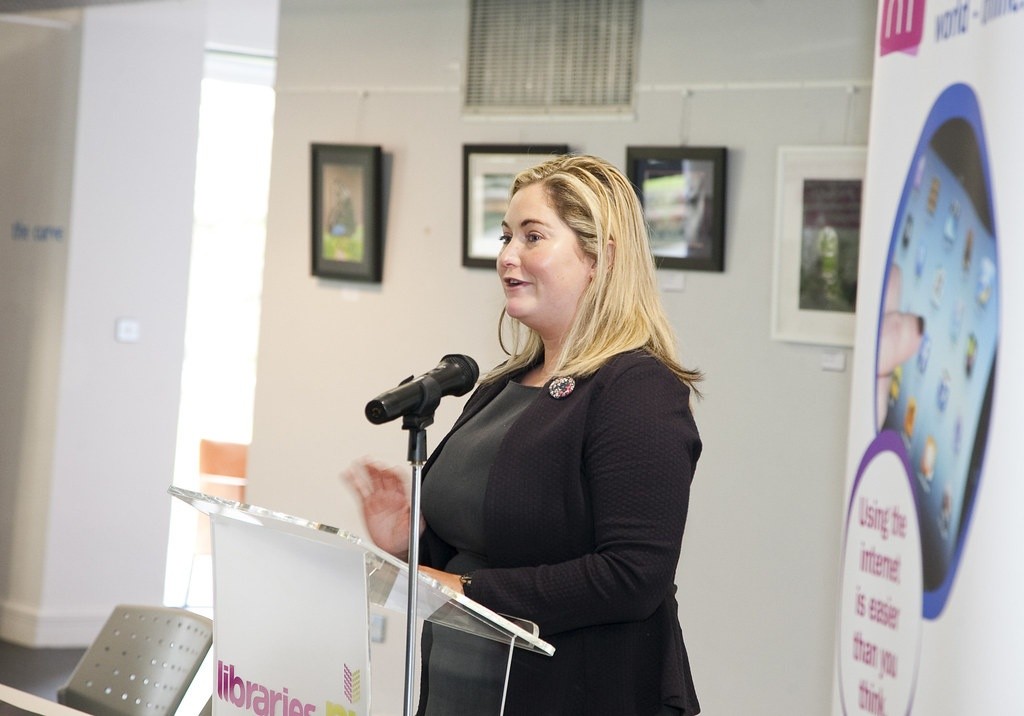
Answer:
[365,354,479,426]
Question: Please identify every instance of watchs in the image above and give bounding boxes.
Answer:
[459,570,476,598]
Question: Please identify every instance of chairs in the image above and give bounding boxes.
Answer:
[56,604,214,716]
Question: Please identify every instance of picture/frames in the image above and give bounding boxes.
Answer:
[770,143,866,348]
[309,141,382,283]
[463,143,570,268]
[623,146,728,272]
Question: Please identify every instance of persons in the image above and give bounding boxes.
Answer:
[341,152,702,716]
